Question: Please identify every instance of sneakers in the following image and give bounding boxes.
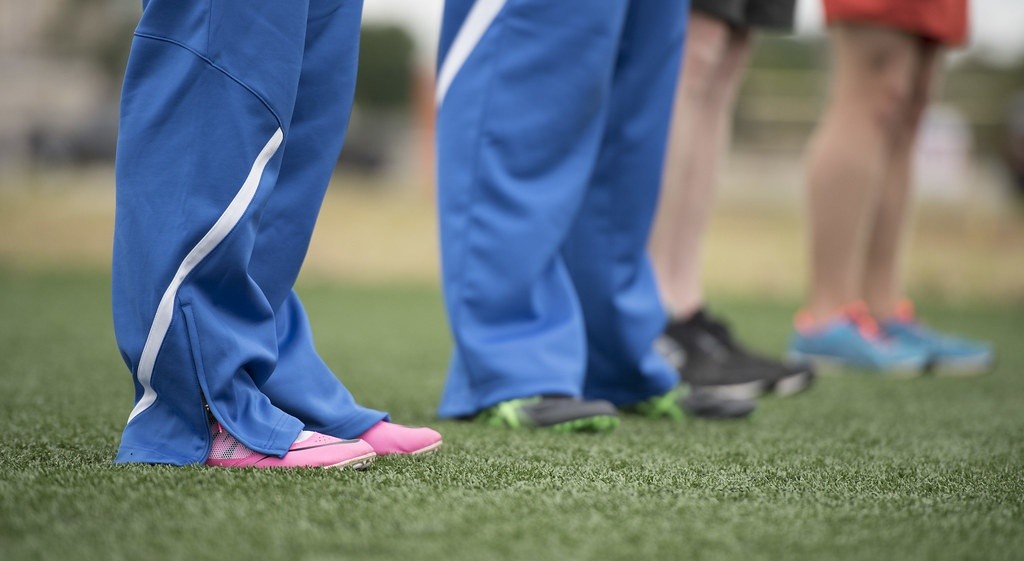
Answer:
[473,393,618,433]
[879,299,995,378]
[784,303,927,379]
[620,384,753,424]
[360,422,444,459]
[204,415,377,471]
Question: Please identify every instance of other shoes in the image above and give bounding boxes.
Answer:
[665,312,812,414]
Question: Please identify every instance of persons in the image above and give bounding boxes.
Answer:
[790,0,997,375]
[111,0,443,471]
[433,0,755,435]
[649,0,814,419]
